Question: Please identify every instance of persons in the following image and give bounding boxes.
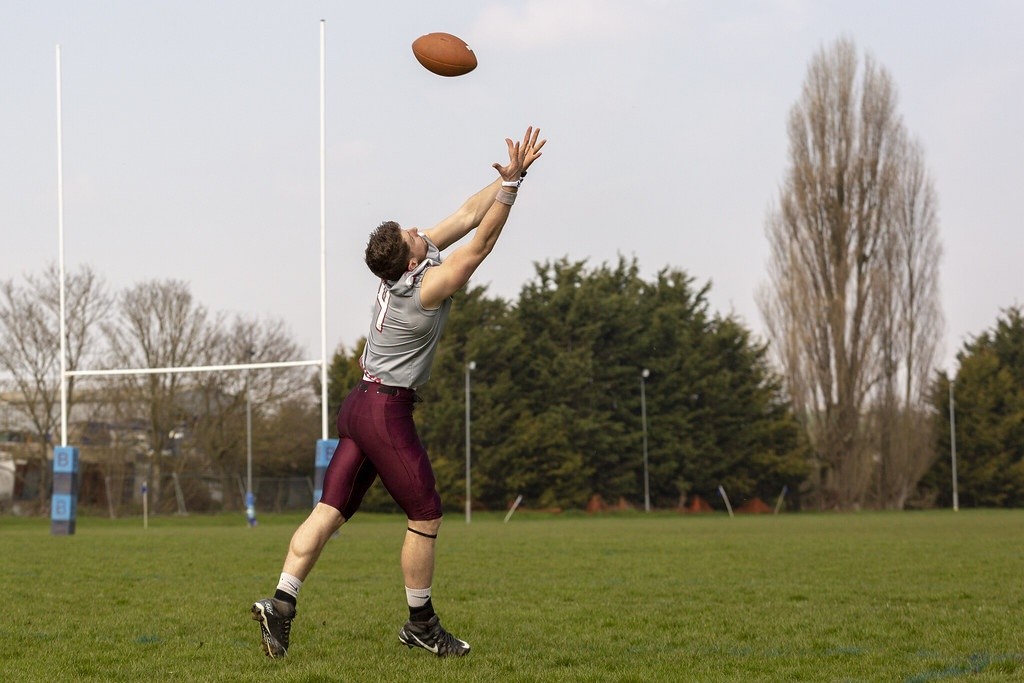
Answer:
[250,126,546,658]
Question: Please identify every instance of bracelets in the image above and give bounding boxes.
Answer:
[499,180,521,188]
[495,188,518,205]
[519,172,528,182]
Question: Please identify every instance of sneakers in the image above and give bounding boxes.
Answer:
[397,612,471,657]
[251,598,297,660]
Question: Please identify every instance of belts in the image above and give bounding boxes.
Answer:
[360,384,397,395]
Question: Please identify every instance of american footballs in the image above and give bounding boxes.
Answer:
[411,32,478,78]
